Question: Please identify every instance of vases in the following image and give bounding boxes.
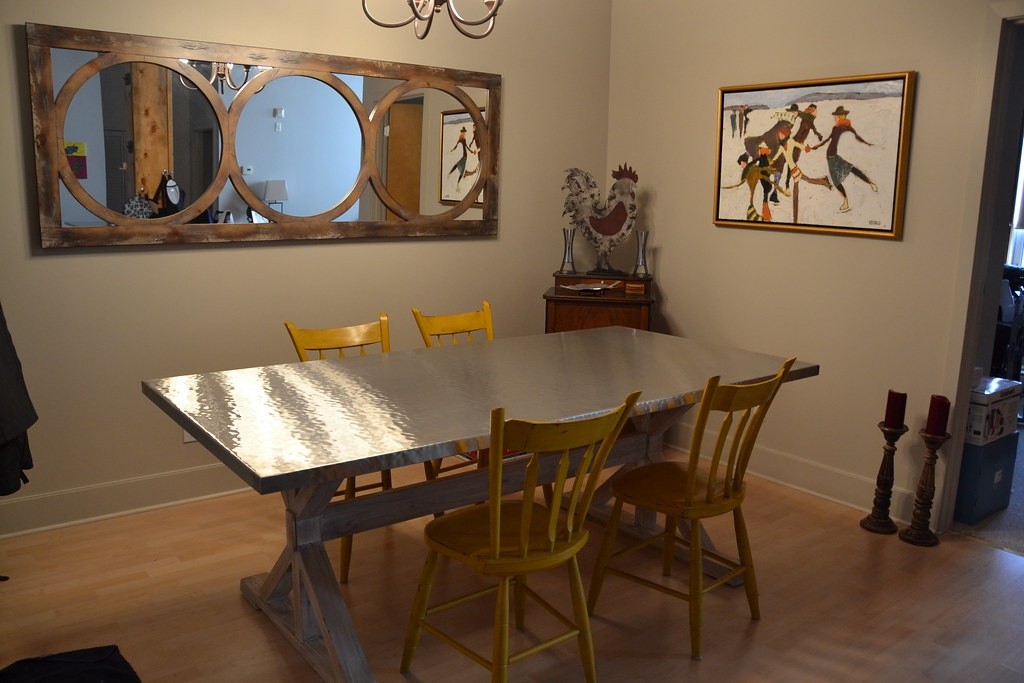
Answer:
[558,228,576,274]
[633,229,649,276]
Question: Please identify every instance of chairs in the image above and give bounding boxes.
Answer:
[283,314,393,584]
[588,358,797,661]
[411,300,493,519]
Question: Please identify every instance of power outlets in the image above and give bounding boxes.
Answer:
[183,429,197,443]
[276,122,282,132]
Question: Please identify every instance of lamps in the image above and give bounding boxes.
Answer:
[265,180,288,223]
[179,58,274,94]
[362,0,504,40]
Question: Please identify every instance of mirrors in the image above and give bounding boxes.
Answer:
[25,21,502,240]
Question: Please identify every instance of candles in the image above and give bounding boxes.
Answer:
[884,389,907,429]
[925,394,950,437]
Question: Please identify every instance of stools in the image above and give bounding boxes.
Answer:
[399,391,641,683]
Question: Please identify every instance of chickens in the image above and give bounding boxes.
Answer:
[560,161,640,276]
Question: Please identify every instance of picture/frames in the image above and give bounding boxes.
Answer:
[713,71,917,242]
[438,106,485,208]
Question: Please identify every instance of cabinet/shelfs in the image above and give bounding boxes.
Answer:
[543,271,655,334]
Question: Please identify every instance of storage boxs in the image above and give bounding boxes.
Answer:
[953,430,1020,525]
[964,376,1023,447]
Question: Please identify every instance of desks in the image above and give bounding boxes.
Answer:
[141,326,820,683]
